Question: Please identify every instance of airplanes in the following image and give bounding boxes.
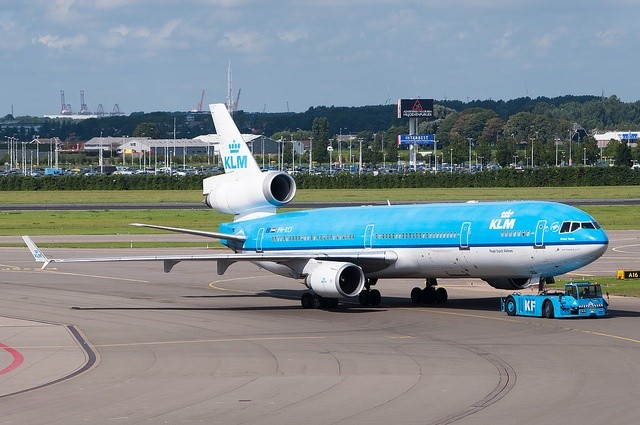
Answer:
[24,105,608,313]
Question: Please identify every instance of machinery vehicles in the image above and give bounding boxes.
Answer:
[501,282,610,319]
